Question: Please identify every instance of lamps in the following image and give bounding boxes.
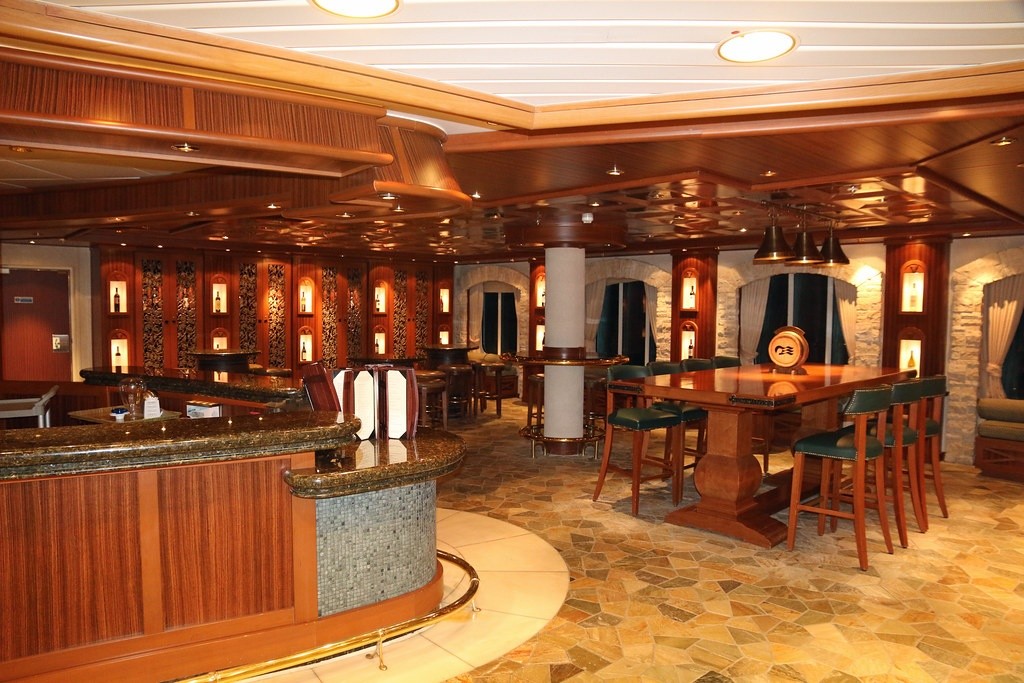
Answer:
[812,236,850,267]
[785,231,825,265]
[752,225,798,262]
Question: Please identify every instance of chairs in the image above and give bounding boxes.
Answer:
[679,355,772,480]
[786,382,895,572]
[592,364,681,517]
[630,361,709,500]
[867,374,949,531]
[829,378,926,549]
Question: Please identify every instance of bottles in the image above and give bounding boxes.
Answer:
[688,339,693,359]
[215,291,220,312]
[542,332,545,351]
[302,342,306,360]
[115,346,121,374]
[690,286,695,309]
[440,296,443,312]
[375,294,380,311]
[911,283,917,311]
[541,289,545,306]
[375,339,379,354]
[908,350,915,369]
[115,288,119,312]
[301,292,306,312]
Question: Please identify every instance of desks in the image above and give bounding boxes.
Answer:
[498,350,630,460]
[184,349,263,374]
[346,353,428,369]
[415,343,480,421]
[608,362,919,549]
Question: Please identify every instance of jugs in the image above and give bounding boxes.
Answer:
[118,377,155,416]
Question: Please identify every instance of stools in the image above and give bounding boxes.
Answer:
[518,373,545,437]
[437,363,475,427]
[248,363,264,374]
[265,367,293,378]
[414,368,448,433]
[473,360,506,419]
